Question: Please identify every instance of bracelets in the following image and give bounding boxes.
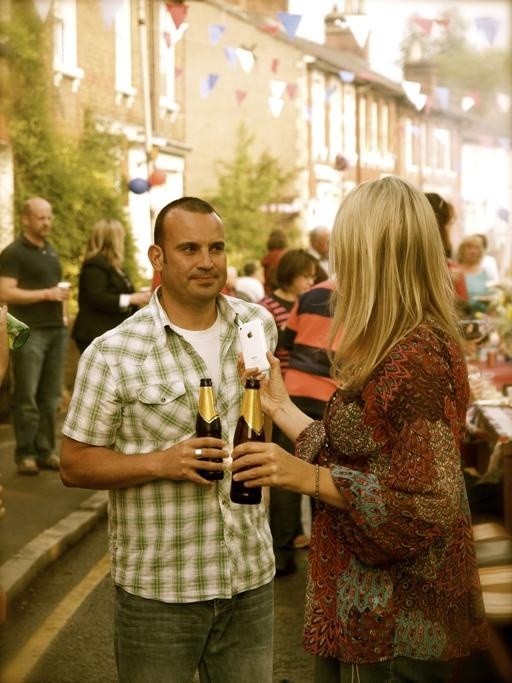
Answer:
[315,464,319,503]
[43,289,49,300]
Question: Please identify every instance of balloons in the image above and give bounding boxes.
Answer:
[128,178,151,195]
[148,169,168,189]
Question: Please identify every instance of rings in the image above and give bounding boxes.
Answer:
[195,448,202,460]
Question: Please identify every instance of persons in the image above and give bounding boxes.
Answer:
[271,273,344,581]
[0,302,8,380]
[230,176,493,682]
[71,218,153,354]
[456,235,497,314]
[256,249,317,547]
[307,226,330,287]
[424,192,455,260]
[473,233,496,268]
[0,196,70,474]
[261,228,288,296]
[236,260,266,284]
[58,196,279,683]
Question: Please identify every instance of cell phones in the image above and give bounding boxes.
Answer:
[238,317,272,374]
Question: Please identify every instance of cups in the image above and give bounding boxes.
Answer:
[59,281,71,302]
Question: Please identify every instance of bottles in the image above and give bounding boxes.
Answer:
[231,381,269,505]
[194,377,226,481]
